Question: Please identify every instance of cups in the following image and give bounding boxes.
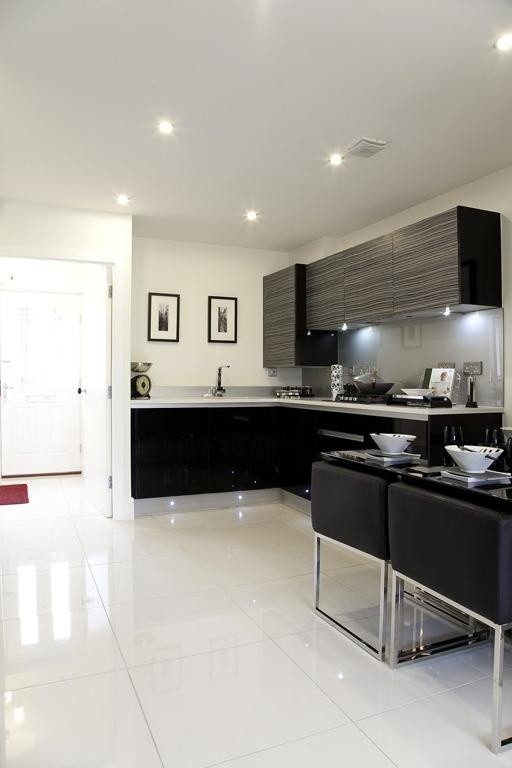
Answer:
[485,428,502,448]
[444,426,465,470]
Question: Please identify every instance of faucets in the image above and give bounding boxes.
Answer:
[207,362,231,398]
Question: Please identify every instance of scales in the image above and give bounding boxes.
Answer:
[130,362,154,400]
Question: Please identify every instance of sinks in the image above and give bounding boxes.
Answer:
[205,396,250,400]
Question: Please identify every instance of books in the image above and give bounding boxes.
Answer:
[428,368,455,400]
[440,467,511,484]
[363,449,420,462]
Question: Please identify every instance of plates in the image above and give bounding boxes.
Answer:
[401,389,434,396]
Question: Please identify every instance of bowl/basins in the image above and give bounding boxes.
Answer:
[369,433,417,455]
[445,445,504,474]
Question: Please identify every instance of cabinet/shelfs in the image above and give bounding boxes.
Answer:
[131,407,278,500]
[305,206,503,333]
[280,406,505,502]
[261,263,339,369]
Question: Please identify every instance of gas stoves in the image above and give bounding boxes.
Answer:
[337,393,393,403]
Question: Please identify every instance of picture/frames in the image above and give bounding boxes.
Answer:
[207,295,238,344]
[147,292,180,342]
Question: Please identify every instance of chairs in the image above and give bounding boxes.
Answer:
[426,410,504,462]
[310,453,488,667]
[386,477,512,754]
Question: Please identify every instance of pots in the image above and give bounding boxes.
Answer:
[353,379,395,395]
